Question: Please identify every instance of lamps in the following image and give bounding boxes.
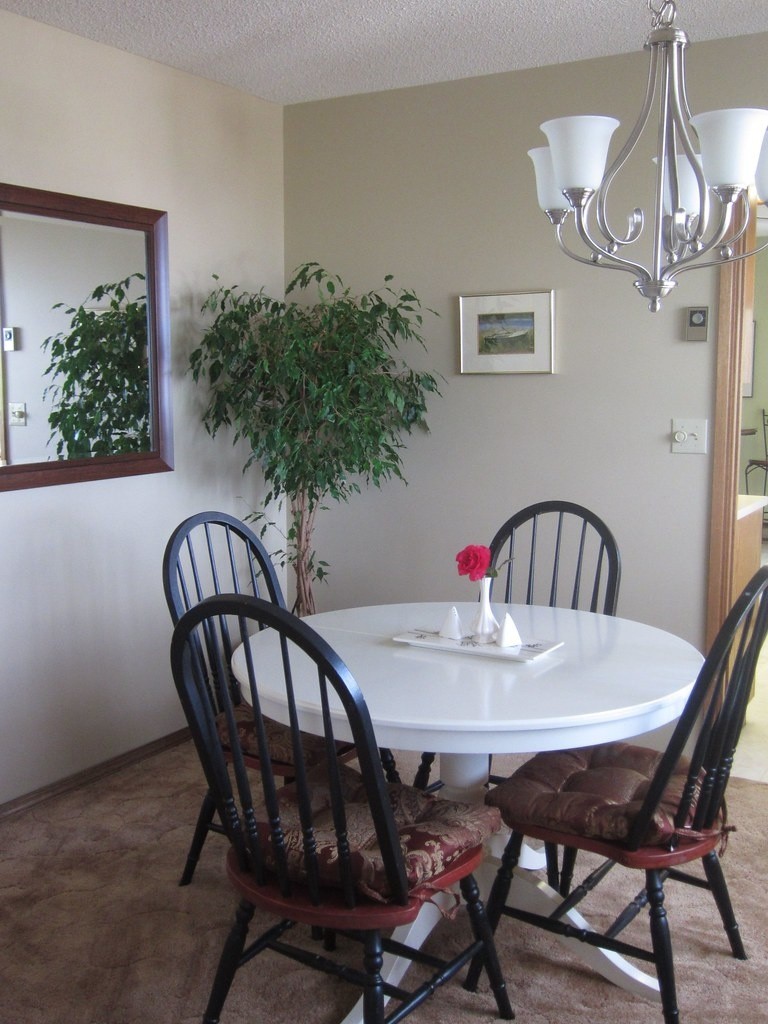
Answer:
[527,0,768,315]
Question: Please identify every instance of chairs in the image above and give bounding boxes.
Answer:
[413,498,628,901]
[466,566,768,1024]
[169,590,515,1024]
[163,509,402,890]
[745,408,768,541]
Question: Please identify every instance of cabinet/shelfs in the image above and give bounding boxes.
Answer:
[728,494,768,701]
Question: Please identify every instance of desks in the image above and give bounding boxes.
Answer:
[230,600,706,1024]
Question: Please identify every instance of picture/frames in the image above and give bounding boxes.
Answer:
[457,289,556,377]
[743,319,756,398]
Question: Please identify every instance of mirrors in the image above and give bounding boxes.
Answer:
[0,183,176,495]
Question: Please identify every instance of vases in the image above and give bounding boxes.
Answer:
[469,576,500,646]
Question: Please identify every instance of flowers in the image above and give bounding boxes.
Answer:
[455,544,515,582]
